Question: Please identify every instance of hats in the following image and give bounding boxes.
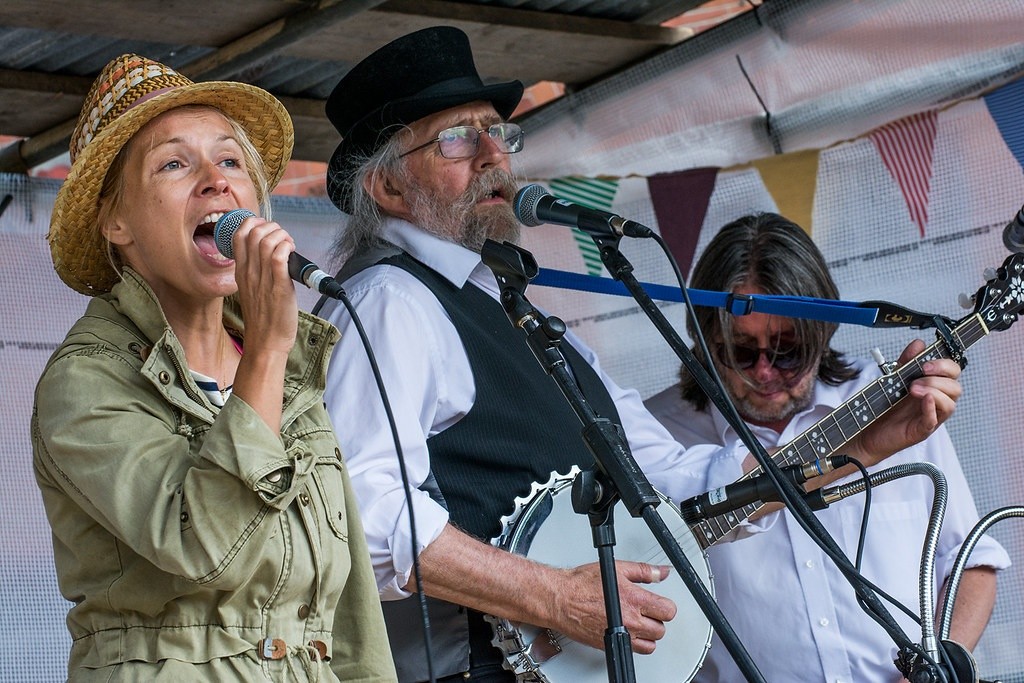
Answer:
[324,24,524,215]
[47,54,295,298]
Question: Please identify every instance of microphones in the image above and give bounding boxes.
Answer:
[214,208,347,299]
[514,183,650,238]
[1002,205,1024,253]
[681,454,849,522]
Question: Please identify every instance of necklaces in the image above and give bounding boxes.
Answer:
[223,327,227,402]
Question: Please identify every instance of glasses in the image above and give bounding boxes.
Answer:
[706,335,810,371]
[388,123,525,162]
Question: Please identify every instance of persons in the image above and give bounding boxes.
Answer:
[29,53,397,683]
[321,24,966,683]
[644,212,1011,683]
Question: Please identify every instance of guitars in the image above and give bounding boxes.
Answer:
[484,253,1024,683]
